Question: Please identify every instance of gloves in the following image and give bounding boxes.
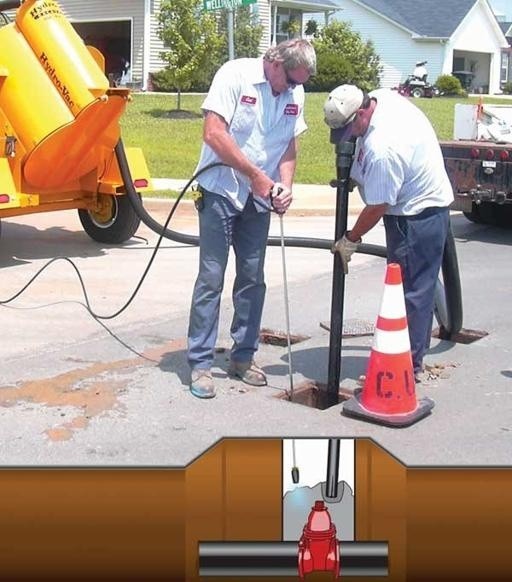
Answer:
[331,231,361,274]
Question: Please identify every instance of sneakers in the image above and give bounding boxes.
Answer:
[191,369,215,398]
[228,361,267,385]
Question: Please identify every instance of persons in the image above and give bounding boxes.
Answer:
[187,39,317,399]
[324,84,454,385]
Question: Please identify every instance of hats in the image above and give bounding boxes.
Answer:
[323,84,364,144]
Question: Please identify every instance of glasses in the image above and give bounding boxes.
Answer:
[286,70,303,87]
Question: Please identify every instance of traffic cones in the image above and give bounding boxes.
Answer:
[339,261,436,429]
